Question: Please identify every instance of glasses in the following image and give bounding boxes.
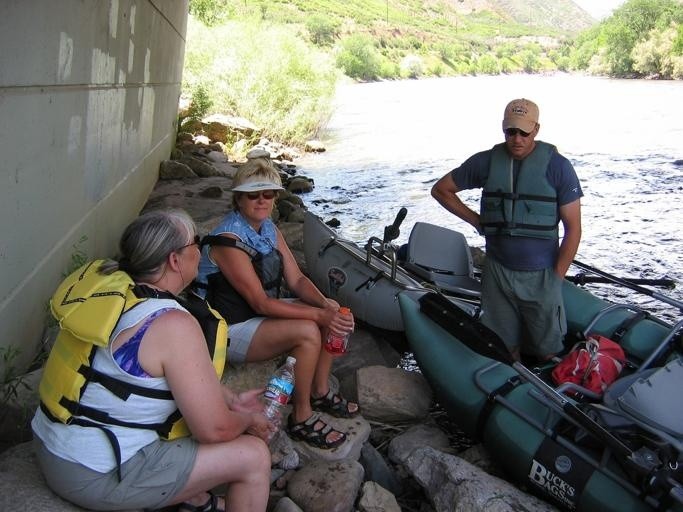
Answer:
[246,190,275,200]
[506,128,530,138]
[180,236,201,249]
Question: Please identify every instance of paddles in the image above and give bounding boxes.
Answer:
[420,291,683,503]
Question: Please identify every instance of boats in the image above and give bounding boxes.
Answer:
[396,268,682,512]
[298,205,490,332]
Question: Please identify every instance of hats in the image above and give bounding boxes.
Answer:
[231,176,285,192]
[503,98,539,133]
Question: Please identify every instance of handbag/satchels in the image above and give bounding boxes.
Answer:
[552,335,624,399]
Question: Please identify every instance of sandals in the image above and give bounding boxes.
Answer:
[289,415,345,448]
[311,389,360,418]
[175,489,225,509]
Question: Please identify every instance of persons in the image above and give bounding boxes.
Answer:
[431,100,582,377]
[188,149,360,444]
[30,206,271,511]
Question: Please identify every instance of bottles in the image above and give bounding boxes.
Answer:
[247,356,297,436]
[324,306,351,355]
